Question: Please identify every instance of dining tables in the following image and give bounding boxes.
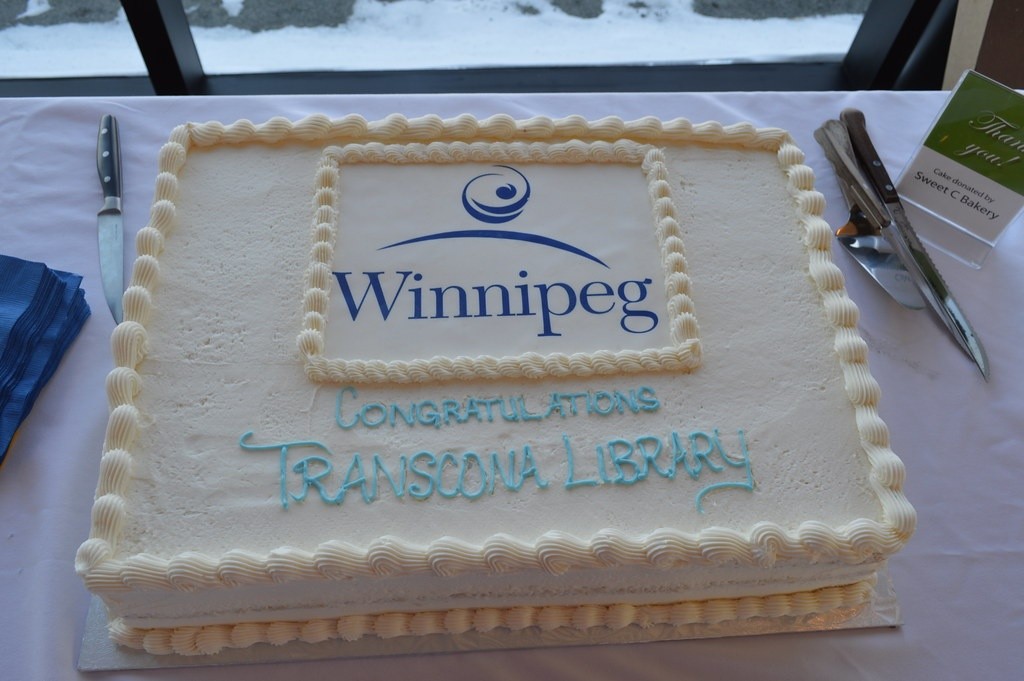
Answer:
[1,90,1024,681]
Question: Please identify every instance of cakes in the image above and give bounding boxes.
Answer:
[75,110,919,652]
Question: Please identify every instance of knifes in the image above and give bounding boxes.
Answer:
[812,124,978,367]
[95,114,125,325]
[842,106,993,384]
